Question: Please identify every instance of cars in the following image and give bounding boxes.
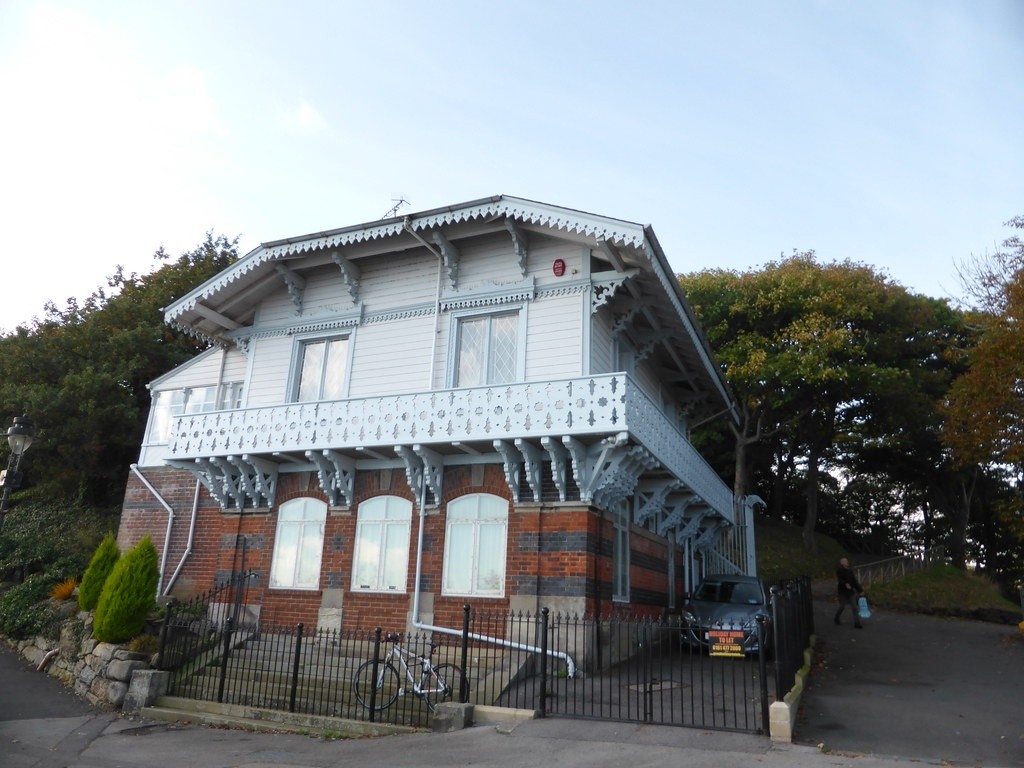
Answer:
[680,574,774,656]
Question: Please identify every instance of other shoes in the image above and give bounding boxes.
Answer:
[855,622,863,630]
[834,615,840,625]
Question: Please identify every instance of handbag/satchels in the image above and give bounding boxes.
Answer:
[857,598,872,618]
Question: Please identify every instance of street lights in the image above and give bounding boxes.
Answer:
[0,414,37,528]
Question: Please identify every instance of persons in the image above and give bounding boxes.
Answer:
[834,557,865,629]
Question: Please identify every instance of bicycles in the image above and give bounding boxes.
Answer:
[351,632,471,717]
